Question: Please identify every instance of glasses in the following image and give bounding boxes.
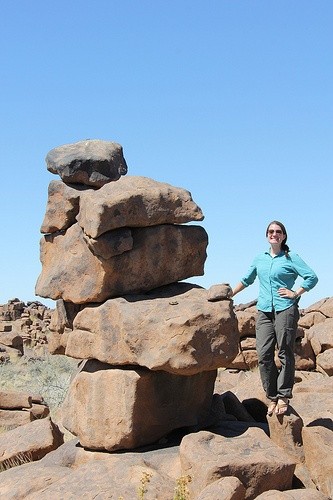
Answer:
[268,230,282,234]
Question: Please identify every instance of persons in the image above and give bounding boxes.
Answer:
[232,220,318,417]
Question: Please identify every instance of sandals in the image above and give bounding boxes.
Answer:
[275,398,289,416]
[267,402,277,415]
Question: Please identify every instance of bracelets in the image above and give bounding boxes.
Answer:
[293,292,298,297]
